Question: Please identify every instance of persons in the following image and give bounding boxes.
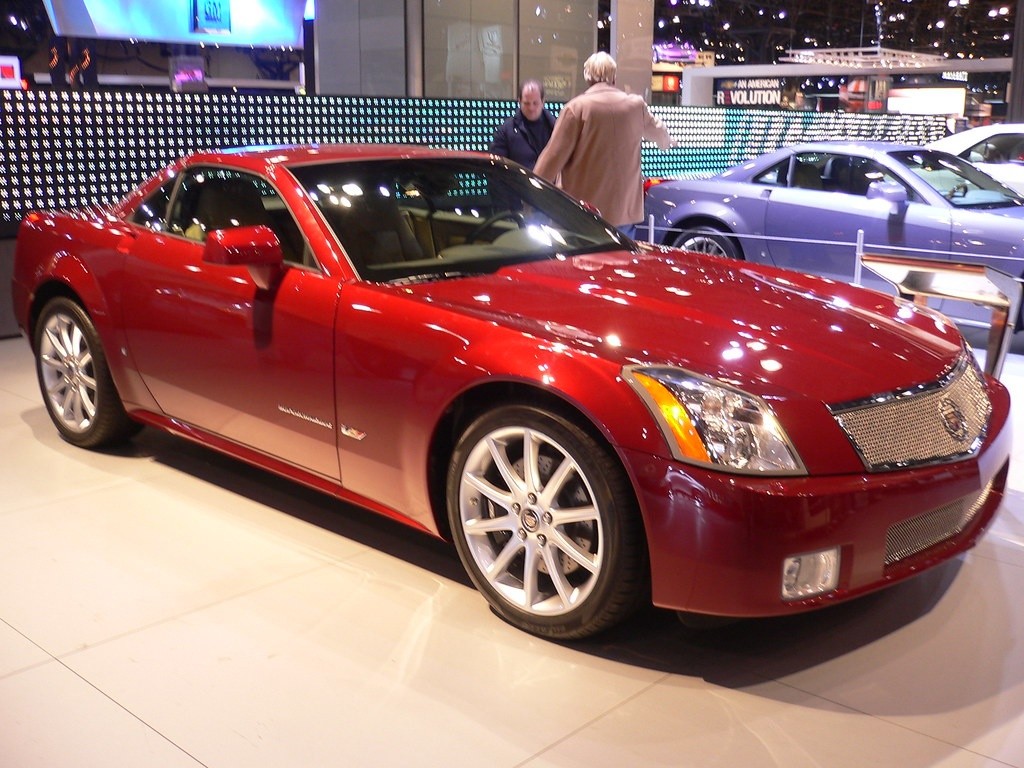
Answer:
[485,79,557,223]
[523,51,678,241]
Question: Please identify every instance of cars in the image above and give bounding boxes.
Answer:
[652,40,697,64]
[882,123,1024,205]
[635,141,1024,333]
[11,144,1013,640]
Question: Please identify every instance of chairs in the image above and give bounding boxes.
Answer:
[194,177,273,242]
[794,163,824,191]
[825,157,853,193]
[337,190,425,265]
